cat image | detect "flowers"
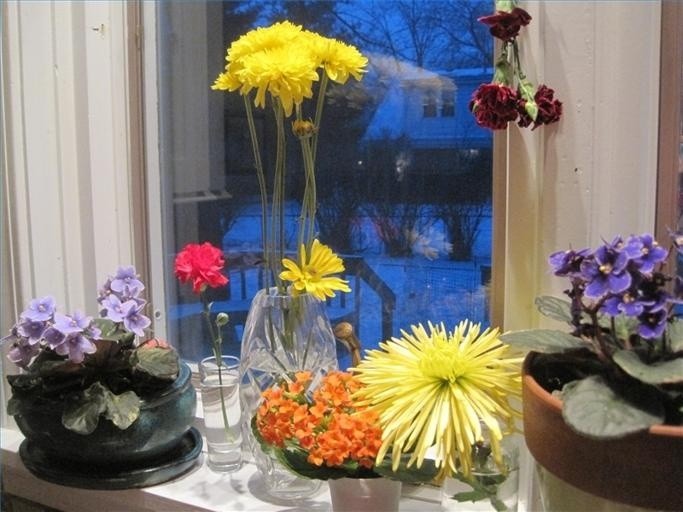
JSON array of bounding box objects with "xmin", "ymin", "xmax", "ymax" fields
[
  {"xmin": 255, "ymin": 320, "xmax": 521, "ymax": 480},
  {"xmin": 5, "ymin": 263, "xmax": 180, "ymax": 433},
  {"xmin": 211, "ymin": 18, "xmax": 371, "ymax": 383},
  {"xmin": 463, "ymin": 0, "xmax": 563, "ymax": 132},
  {"xmin": 498, "ymin": 217, "xmax": 682, "ymax": 442},
  {"xmin": 175, "ymin": 241, "xmax": 243, "ymax": 456}
]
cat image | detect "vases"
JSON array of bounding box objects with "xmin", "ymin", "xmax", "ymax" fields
[
  {"xmin": 242, "ymin": 283, "xmax": 340, "ymax": 394},
  {"xmin": 522, "ymin": 366, "xmax": 681, "ymax": 511},
  {"xmin": 9, "ymin": 357, "xmax": 193, "ymax": 454},
  {"xmin": 330, "ymin": 476, "xmax": 402, "ymax": 511},
  {"xmin": 440, "ymin": 416, "xmax": 519, "ymax": 510}
]
